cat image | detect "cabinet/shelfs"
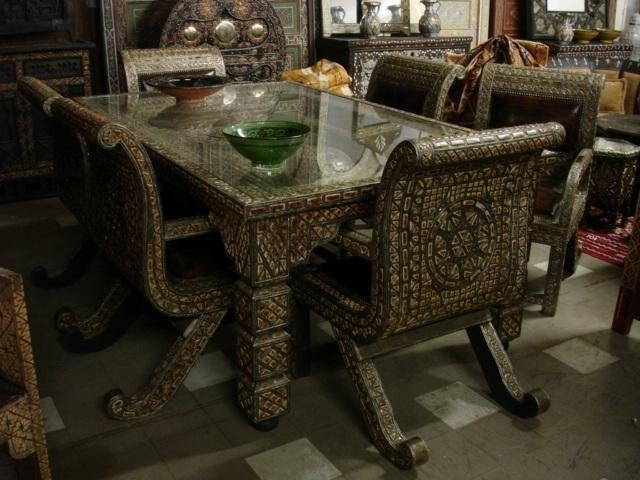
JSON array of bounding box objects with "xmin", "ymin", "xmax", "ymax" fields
[
  {"xmin": 316, "ymin": 38, "xmax": 473, "ymax": 98},
  {"xmin": 0, "ymin": 38, "xmax": 93, "ymax": 183}
]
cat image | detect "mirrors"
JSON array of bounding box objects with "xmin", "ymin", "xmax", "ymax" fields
[{"xmin": 319, "ymin": 0, "xmax": 411, "ymax": 37}]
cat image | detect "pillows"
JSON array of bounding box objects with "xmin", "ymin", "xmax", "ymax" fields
[{"xmin": 600, "ymin": 78, "xmax": 627, "ymax": 113}]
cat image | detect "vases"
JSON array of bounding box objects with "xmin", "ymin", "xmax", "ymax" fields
[
  {"xmin": 332, "ymin": 7, "xmax": 345, "ymax": 23},
  {"xmin": 557, "ymin": 15, "xmax": 574, "ymax": 46},
  {"xmin": 386, "ymin": 6, "xmax": 402, "ymax": 23},
  {"xmin": 419, "ymin": 0, "xmax": 441, "ymax": 37},
  {"xmin": 361, "ymin": 2, "xmax": 380, "ymax": 39}
]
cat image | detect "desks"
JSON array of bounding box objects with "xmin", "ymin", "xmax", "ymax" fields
[{"xmin": 544, "ymin": 41, "xmax": 634, "ymax": 60}]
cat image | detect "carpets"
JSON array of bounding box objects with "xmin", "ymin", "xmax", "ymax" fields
[{"xmin": 539, "ymin": 190, "xmax": 635, "ymax": 266}]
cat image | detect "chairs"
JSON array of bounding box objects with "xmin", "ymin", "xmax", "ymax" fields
[
  {"xmin": 285, "ymin": 122, "xmax": 565, "ymax": 471},
  {"xmin": 612, "ymin": 191, "xmax": 640, "ymax": 335},
  {"xmin": 0, "ymin": 267, "xmax": 52, "ymax": 479},
  {"xmin": 19, "ymin": 45, "xmax": 605, "ymax": 432}
]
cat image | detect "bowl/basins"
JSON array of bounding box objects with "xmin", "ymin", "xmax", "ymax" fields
[
  {"xmin": 573, "ymin": 28, "xmax": 621, "ymax": 45},
  {"xmin": 146, "ymin": 75, "xmax": 231, "ymax": 104},
  {"xmin": 223, "ymin": 120, "xmax": 312, "ymax": 169}
]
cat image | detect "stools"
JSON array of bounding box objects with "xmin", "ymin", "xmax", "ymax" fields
[{"xmin": 586, "ymin": 137, "xmax": 640, "ymax": 227}]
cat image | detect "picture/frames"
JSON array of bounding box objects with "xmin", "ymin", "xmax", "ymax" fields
[{"xmin": 526, "ymin": 0, "xmax": 609, "ymax": 39}]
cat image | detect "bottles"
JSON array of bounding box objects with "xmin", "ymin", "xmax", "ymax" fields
[
  {"xmin": 556, "ymin": 12, "xmax": 575, "ymax": 46},
  {"xmin": 419, "ymin": 0, "xmax": 441, "ymax": 39},
  {"xmin": 330, "ymin": 6, "xmax": 345, "ymax": 25},
  {"xmin": 361, "ymin": 1, "xmax": 382, "ymax": 40},
  {"xmin": 386, "ymin": 5, "xmax": 403, "ymax": 24}
]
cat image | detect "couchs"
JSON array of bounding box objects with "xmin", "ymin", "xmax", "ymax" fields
[{"xmin": 548, "ymin": 56, "xmax": 640, "ymax": 116}]
